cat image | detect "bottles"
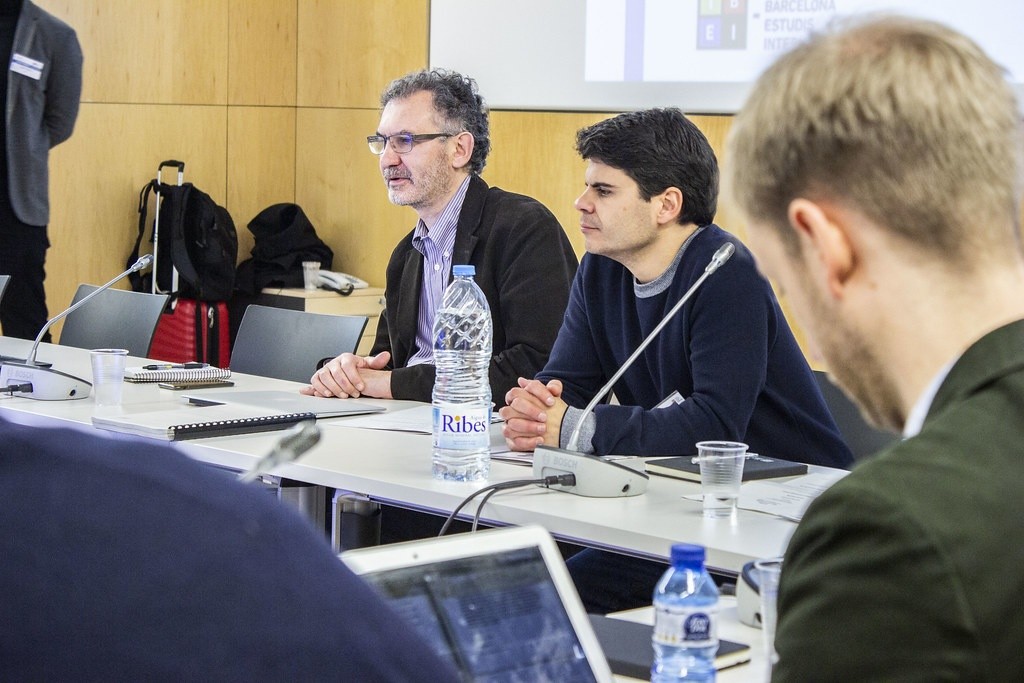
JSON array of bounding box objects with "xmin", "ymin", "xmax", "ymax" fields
[
  {"xmin": 432, "ymin": 265, "xmax": 491, "ymax": 483},
  {"xmin": 651, "ymin": 544, "xmax": 719, "ymax": 683}
]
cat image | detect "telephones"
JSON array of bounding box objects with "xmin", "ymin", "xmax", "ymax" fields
[{"xmin": 311, "ymin": 270, "xmax": 369, "ymax": 290}]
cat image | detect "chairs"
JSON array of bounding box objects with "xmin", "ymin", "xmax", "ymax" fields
[
  {"xmin": 230, "ymin": 305, "xmax": 370, "ymax": 390},
  {"xmin": 58, "ymin": 284, "xmax": 171, "ymax": 358}
]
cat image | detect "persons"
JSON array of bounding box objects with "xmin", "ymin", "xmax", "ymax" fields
[
  {"xmin": 498, "ymin": 108, "xmax": 858, "ymax": 617},
  {"xmin": 0, "ymin": 415, "xmax": 458, "ymax": 683},
  {"xmin": 0, "ymin": 0, "xmax": 83, "ymax": 345},
  {"xmin": 299, "ymin": 67, "xmax": 579, "ymax": 545},
  {"xmin": 727, "ymin": 13, "xmax": 1024, "ymax": 683}
]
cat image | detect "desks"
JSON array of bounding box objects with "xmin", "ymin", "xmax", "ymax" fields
[
  {"xmin": 0, "ymin": 336, "xmax": 852, "ymax": 580},
  {"xmin": 262, "ymin": 287, "xmax": 390, "ymax": 356},
  {"xmin": 602, "ymin": 595, "xmax": 764, "ymax": 683}
]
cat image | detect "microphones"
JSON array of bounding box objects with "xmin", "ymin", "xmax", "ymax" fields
[
  {"xmin": 0, "ymin": 254, "xmax": 157, "ymax": 400},
  {"xmin": 534, "ymin": 243, "xmax": 736, "ymax": 497}
]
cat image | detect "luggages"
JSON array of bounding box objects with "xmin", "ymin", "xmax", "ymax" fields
[{"xmin": 146, "ymin": 160, "xmax": 229, "ymax": 373}]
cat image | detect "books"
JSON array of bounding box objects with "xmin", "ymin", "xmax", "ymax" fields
[
  {"xmin": 587, "ymin": 614, "xmax": 752, "ymax": 682},
  {"xmin": 125, "ymin": 360, "xmax": 235, "ymax": 383},
  {"xmin": 644, "ymin": 455, "xmax": 808, "ymax": 485},
  {"xmin": 92, "ymin": 402, "xmax": 316, "ymax": 442}
]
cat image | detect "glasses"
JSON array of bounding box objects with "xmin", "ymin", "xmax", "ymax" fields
[{"xmin": 367, "ymin": 133, "xmax": 453, "ymax": 155}]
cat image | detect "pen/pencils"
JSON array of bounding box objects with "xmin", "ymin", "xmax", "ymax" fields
[
  {"xmin": 691, "ymin": 452, "xmax": 759, "ymax": 464},
  {"xmin": 143, "ymin": 363, "xmax": 211, "ymax": 370}
]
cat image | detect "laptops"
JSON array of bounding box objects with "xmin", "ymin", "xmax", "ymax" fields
[{"xmin": 336, "ymin": 523, "xmax": 615, "ymax": 683}]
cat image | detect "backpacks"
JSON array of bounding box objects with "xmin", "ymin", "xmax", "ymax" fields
[{"xmin": 127, "ymin": 179, "xmax": 236, "ymax": 303}]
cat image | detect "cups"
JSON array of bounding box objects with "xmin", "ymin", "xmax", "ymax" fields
[
  {"xmin": 302, "ymin": 262, "xmax": 320, "ymax": 294},
  {"xmin": 491, "ymin": 402, "xmax": 495, "ymax": 416},
  {"xmin": 90, "ymin": 349, "xmax": 129, "ymax": 406},
  {"xmin": 696, "ymin": 441, "xmax": 749, "ymax": 518},
  {"xmin": 756, "ymin": 558, "xmax": 786, "ymax": 667}
]
